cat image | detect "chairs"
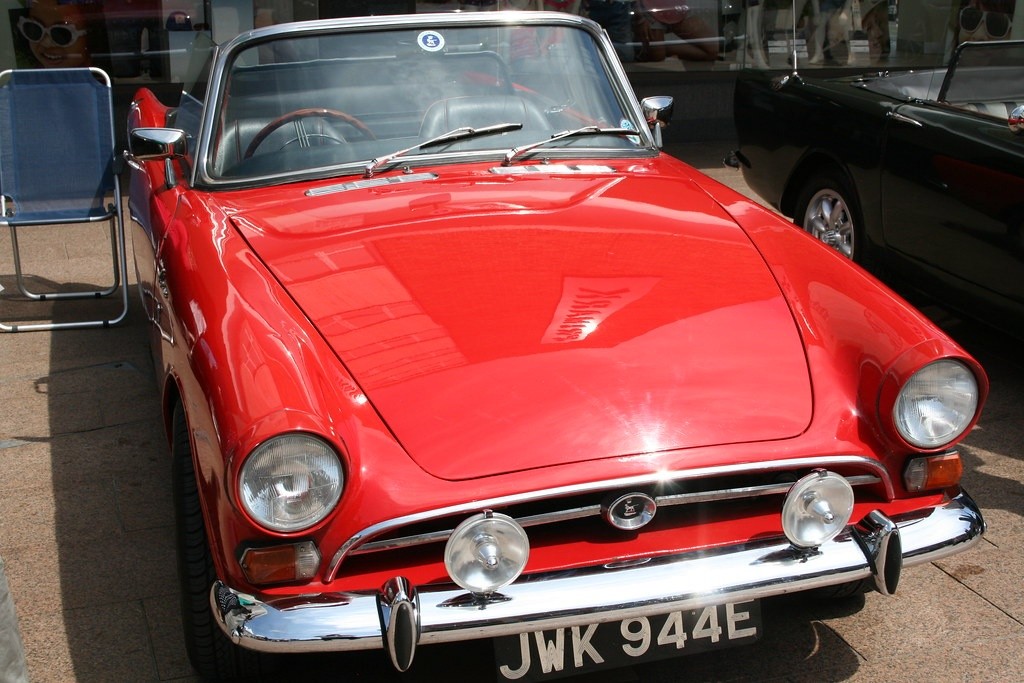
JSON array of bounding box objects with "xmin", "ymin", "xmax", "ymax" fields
[
  {"xmin": 414, "ymin": 95, "xmax": 558, "ymax": 150},
  {"xmin": 213, "ymin": 118, "xmax": 353, "ymax": 175},
  {"xmin": 0, "ymin": 66, "xmax": 129, "ymax": 334}
]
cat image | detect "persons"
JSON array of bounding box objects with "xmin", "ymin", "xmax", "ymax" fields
[
  {"xmin": 951, "ymin": 0, "xmax": 1016, "ymax": 48},
  {"xmin": 416, "ymin": 0, "xmax": 720, "ymax": 63},
  {"xmin": 17, "ymin": 0, "xmax": 90, "ymax": 69},
  {"xmin": 859, "ymin": 0, "xmax": 887, "ymax": 67},
  {"xmin": 736, "ymin": 0, "xmax": 846, "ymax": 70}
]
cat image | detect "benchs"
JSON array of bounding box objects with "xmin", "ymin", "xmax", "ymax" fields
[
  {"xmin": 961, "ymin": 101, "xmax": 1024, "ymax": 119},
  {"xmin": 186, "ymin": 84, "xmax": 538, "ymax": 139}
]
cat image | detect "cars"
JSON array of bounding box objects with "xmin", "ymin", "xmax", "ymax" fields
[
  {"xmin": 127, "ymin": 10, "xmax": 990, "ymax": 682},
  {"xmin": 725, "ymin": 39, "xmax": 1024, "ymax": 348}
]
